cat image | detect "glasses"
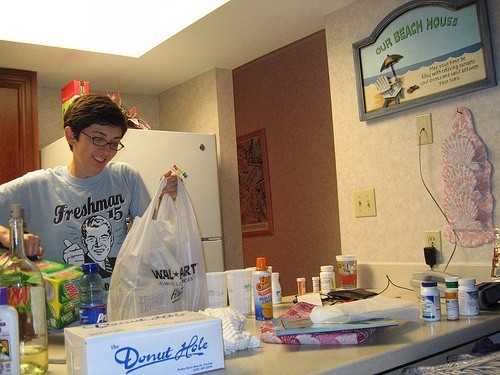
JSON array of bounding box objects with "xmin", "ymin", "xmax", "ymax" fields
[{"xmin": 80, "ymin": 131, "xmax": 124, "ymax": 151}]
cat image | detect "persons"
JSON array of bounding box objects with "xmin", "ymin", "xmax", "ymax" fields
[{"xmin": 0, "ymin": 94, "xmax": 181, "ymax": 334}]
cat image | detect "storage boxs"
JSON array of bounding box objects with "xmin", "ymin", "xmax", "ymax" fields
[{"xmin": 64, "ymin": 310, "xmax": 226, "ymax": 375}]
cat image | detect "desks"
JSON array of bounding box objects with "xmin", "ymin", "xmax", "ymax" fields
[{"xmin": 68, "ymin": 282, "xmax": 500, "ymax": 375}]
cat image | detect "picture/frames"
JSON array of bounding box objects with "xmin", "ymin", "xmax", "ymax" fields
[
  {"xmin": 351, "ymin": 0, "xmax": 500, "ymax": 121},
  {"xmin": 237, "ymin": 128, "xmax": 274, "ymax": 237}
]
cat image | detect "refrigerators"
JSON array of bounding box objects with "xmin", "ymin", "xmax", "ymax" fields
[{"xmin": 40, "ymin": 130, "xmax": 224, "ymax": 273}]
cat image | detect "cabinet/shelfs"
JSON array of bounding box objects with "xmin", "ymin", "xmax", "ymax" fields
[{"xmin": 40, "ymin": 129, "xmax": 226, "ymax": 273}]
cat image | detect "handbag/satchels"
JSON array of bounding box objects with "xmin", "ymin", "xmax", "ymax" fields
[{"xmin": 107, "ymin": 174, "xmax": 209, "ymax": 319}]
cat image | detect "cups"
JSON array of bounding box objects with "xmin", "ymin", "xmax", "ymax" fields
[{"xmin": 206, "ymin": 266, "xmax": 273, "ymax": 317}]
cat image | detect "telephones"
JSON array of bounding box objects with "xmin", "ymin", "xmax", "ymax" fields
[{"xmin": 410, "ymin": 271, "xmax": 464, "ymax": 298}]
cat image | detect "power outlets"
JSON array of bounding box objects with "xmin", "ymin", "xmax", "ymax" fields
[
  {"xmin": 415, "ymin": 113, "xmax": 434, "ymax": 146},
  {"xmin": 425, "ymin": 231, "xmax": 443, "ymax": 264}
]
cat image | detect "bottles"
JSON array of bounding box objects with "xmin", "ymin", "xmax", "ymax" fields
[
  {"xmin": 0, "ymin": 203, "xmax": 49, "ymax": 375},
  {"xmin": 311, "ymin": 277, "xmax": 320, "ymax": 293},
  {"xmin": 445, "ymin": 278, "xmax": 480, "ymax": 320},
  {"xmin": 297, "ymin": 278, "xmax": 306, "ymax": 295},
  {"xmin": 79, "ymin": 263, "xmax": 108, "ymax": 325},
  {"xmin": 272, "ymin": 273, "xmax": 282, "ymax": 304},
  {"xmin": 336, "ymin": 255, "xmax": 358, "ymax": 289},
  {"xmin": 254, "ymin": 257, "xmax": 272, "ymax": 321},
  {"xmin": 320, "ymin": 266, "xmax": 336, "ymax": 292},
  {"xmin": 421, "ymin": 280, "xmax": 441, "ymax": 322}
]
